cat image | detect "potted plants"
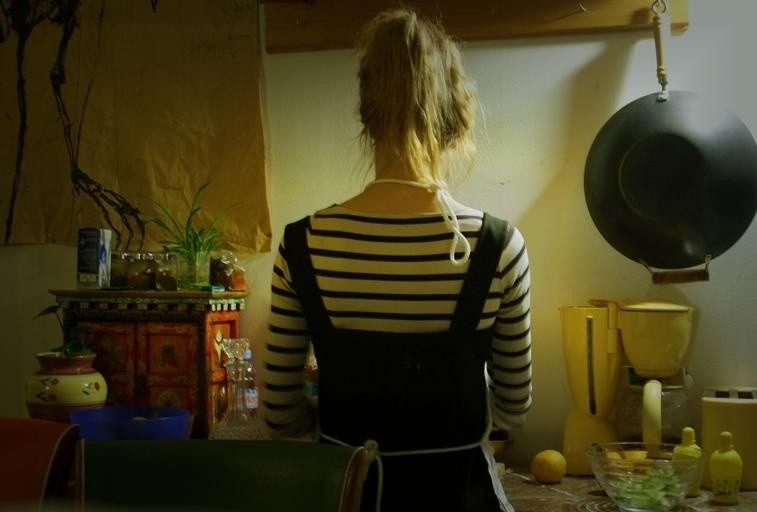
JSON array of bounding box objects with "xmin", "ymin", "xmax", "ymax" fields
[
  {"xmin": 128, "ymin": 180, "xmax": 243, "ymax": 291},
  {"xmin": 25, "ymin": 303, "xmax": 109, "ymax": 423}
]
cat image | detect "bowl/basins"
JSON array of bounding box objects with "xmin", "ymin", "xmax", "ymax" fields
[
  {"xmin": 71, "ymin": 406, "xmax": 189, "ymax": 441},
  {"xmin": 586, "ymin": 441, "xmax": 706, "ymax": 511}
]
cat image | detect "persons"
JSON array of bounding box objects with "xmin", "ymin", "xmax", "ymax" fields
[{"xmin": 258, "ymin": 6, "xmax": 535, "ymax": 511}]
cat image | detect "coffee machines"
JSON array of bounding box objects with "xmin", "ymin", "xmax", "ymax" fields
[{"xmin": 557, "ymin": 301, "xmax": 694, "ymax": 476}]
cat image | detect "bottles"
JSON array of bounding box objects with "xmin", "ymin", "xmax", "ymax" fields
[
  {"xmin": 709, "ymin": 433, "xmax": 743, "ymax": 503},
  {"xmin": 673, "ymin": 426, "xmax": 703, "ymax": 497},
  {"xmin": 112, "ymin": 251, "xmax": 179, "ymax": 292},
  {"xmin": 243, "ymin": 351, "xmax": 259, "ymax": 418}
]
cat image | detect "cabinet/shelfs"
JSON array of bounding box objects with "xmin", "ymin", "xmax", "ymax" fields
[{"xmin": 51, "ymin": 286, "xmax": 249, "ymax": 439}]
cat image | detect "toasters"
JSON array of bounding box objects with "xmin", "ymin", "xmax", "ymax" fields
[{"xmin": 702, "ymin": 387, "xmax": 756, "ymax": 493}]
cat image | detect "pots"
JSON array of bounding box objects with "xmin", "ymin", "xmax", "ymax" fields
[{"xmin": 581, "ymin": 2, "xmax": 756, "ymax": 287}]
